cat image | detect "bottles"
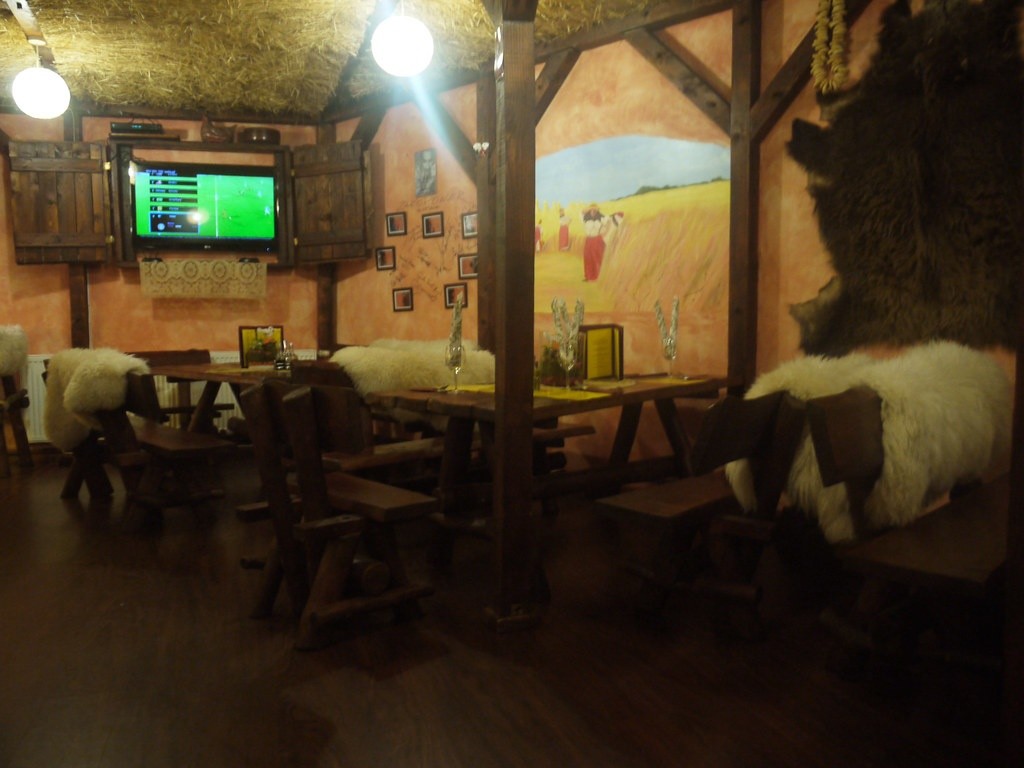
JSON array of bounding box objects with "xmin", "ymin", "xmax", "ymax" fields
[{"xmin": 275, "ymin": 341, "xmax": 299, "ymax": 370}]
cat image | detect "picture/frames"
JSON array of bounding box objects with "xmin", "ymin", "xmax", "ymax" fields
[
  {"xmin": 239, "ymin": 324, "xmax": 284, "ymax": 369},
  {"xmin": 458, "ymin": 254, "xmax": 478, "ymax": 279},
  {"xmin": 422, "ymin": 212, "xmax": 444, "ymax": 238},
  {"xmin": 461, "ymin": 211, "xmax": 478, "ymax": 238},
  {"xmin": 386, "ymin": 212, "xmax": 407, "ymax": 236},
  {"xmin": 392, "ymin": 288, "xmax": 413, "ymax": 312},
  {"xmin": 413, "ymin": 147, "xmax": 437, "ymax": 198},
  {"xmin": 376, "ymin": 246, "xmax": 395, "ymax": 270},
  {"xmin": 444, "ymin": 283, "xmax": 468, "ymax": 308}
]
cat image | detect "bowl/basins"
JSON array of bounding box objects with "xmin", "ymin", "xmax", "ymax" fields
[{"xmin": 242, "ymin": 127, "xmax": 281, "ymax": 145}]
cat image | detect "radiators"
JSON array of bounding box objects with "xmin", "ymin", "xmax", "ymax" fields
[{"xmin": 20, "ymin": 349, "xmax": 317, "ymax": 444}]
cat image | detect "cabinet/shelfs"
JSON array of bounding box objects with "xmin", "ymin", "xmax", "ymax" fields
[{"xmin": 7, "ymin": 139, "xmax": 369, "ymax": 268}]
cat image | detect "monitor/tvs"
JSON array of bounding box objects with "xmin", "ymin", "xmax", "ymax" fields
[{"xmin": 130, "ymin": 160, "xmax": 278, "ymax": 253}]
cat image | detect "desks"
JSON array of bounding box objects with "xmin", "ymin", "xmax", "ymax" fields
[
  {"xmin": 151, "ymin": 363, "xmax": 291, "ymax": 499},
  {"xmin": 364, "ymin": 373, "xmax": 728, "ymax": 577}
]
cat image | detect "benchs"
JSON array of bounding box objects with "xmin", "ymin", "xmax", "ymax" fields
[{"xmin": 0, "ymin": 323, "xmax": 1017, "ymax": 681}]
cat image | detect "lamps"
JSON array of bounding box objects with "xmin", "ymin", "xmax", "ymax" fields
[
  {"xmin": 12, "ymin": 36, "xmax": 70, "ymax": 119},
  {"xmin": 370, "ymin": 1, "xmax": 436, "ymax": 77}
]
